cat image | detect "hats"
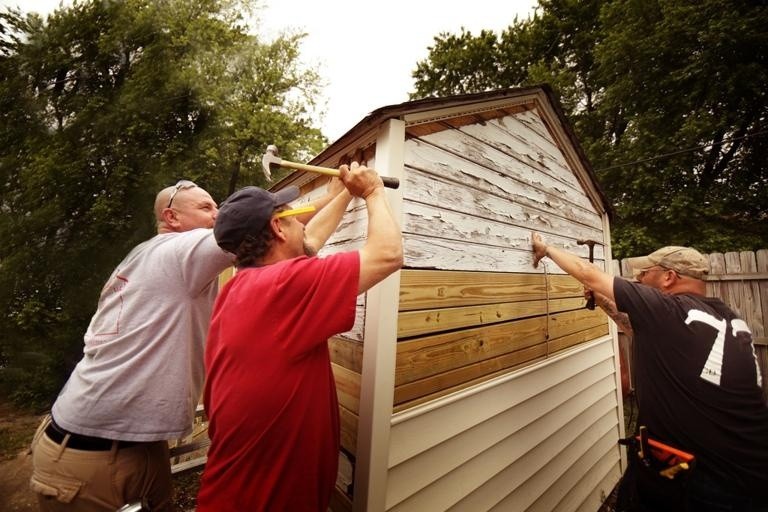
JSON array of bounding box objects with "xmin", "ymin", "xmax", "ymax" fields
[
  {"xmin": 628, "ymin": 244, "xmax": 712, "ymax": 282},
  {"xmin": 214, "ymin": 185, "xmax": 301, "ymax": 253}
]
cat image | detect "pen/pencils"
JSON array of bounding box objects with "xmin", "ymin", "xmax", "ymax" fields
[{"xmin": 271, "ymin": 206, "xmax": 317, "ymax": 219}]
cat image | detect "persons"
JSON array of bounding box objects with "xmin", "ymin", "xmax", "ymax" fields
[
  {"xmin": 29, "ymin": 164, "xmax": 349, "ymax": 512},
  {"xmin": 532, "ymin": 233, "xmax": 768, "ymax": 512},
  {"xmin": 194, "ymin": 160, "xmax": 402, "ymax": 512}
]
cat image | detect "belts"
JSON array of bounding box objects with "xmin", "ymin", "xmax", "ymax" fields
[{"xmin": 44, "ymin": 421, "xmax": 140, "ymax": 451}]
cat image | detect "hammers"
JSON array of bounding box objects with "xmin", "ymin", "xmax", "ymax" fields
[
  {"xmin": 575, "ymin": 237, "xmax": 607, "ymax": 311},
  {"xmin": 261, "ymin": 143, "xmax": 400, "ymax": 190}
]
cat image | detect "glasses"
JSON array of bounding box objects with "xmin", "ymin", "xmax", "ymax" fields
[{"xmin": 168, "ymin": 178, "xmax": 200, "ymax": 210}]
